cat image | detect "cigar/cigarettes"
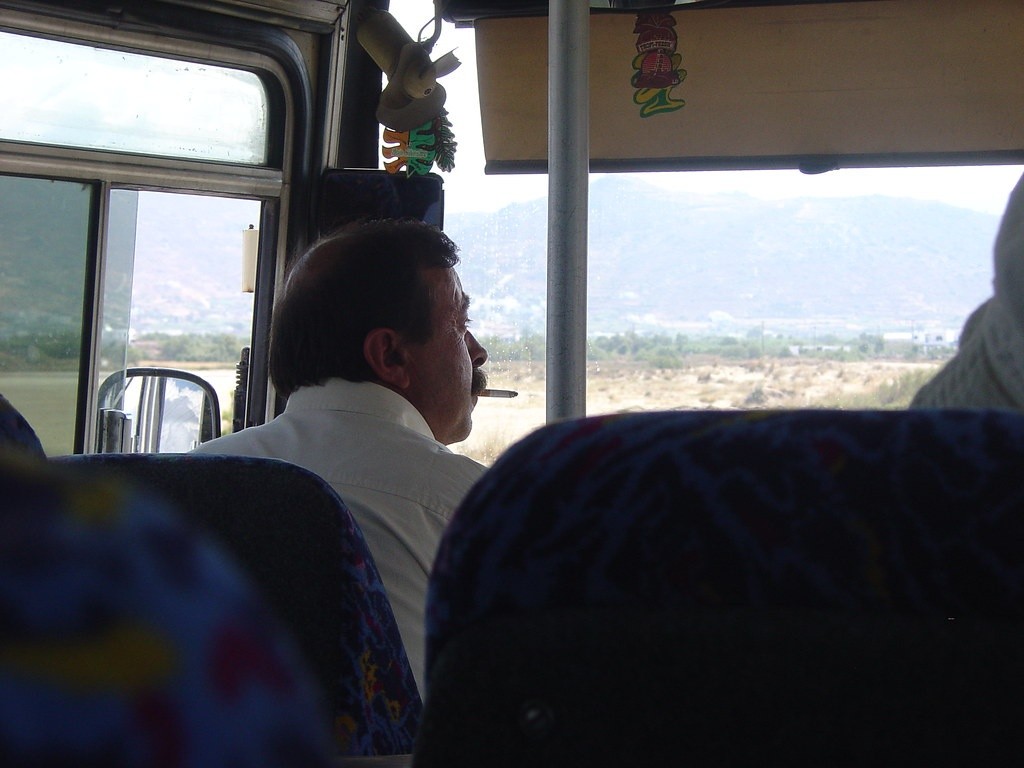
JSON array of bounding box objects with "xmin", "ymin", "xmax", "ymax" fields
[{"xmin": 479, "ymin": 388, "xmax": 518, "ymax": 398}]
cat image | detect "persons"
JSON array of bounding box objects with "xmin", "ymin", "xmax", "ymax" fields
[{"xmin": 185, "ymin": 217, "xmax": 493, "ymax": 707}]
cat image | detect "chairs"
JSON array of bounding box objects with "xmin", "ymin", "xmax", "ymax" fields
[
  {"xmin": 51, "ymin": 452, "xmax": 422, "ymax": 758},
  {"xmin": 416, "ymin": 409, "xmax": 1024, "ymax": 768}
]
